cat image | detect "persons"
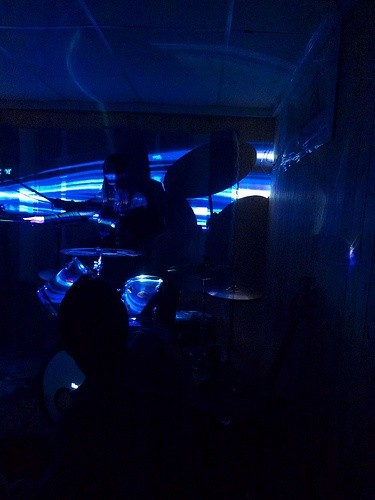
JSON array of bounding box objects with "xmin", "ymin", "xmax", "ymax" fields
[
  {"xmin": 54, "ymin": 276, "xmax": 191, "ymax": 500},
  {"xmin": 51, "ymin": 151, "xmax": 165, "ymax": 252}
]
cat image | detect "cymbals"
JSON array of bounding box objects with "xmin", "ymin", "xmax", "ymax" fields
[
  {"xmin": 57, "ymin": 245, "xmax": 142, "ymax": 258},
  {"xmin": 165, "ymin": 141, "xmax": 257, "ymax": 199},
  {"xmin": 203, "ymin": 281, "xmax": 262, "ymax": 304}
]
